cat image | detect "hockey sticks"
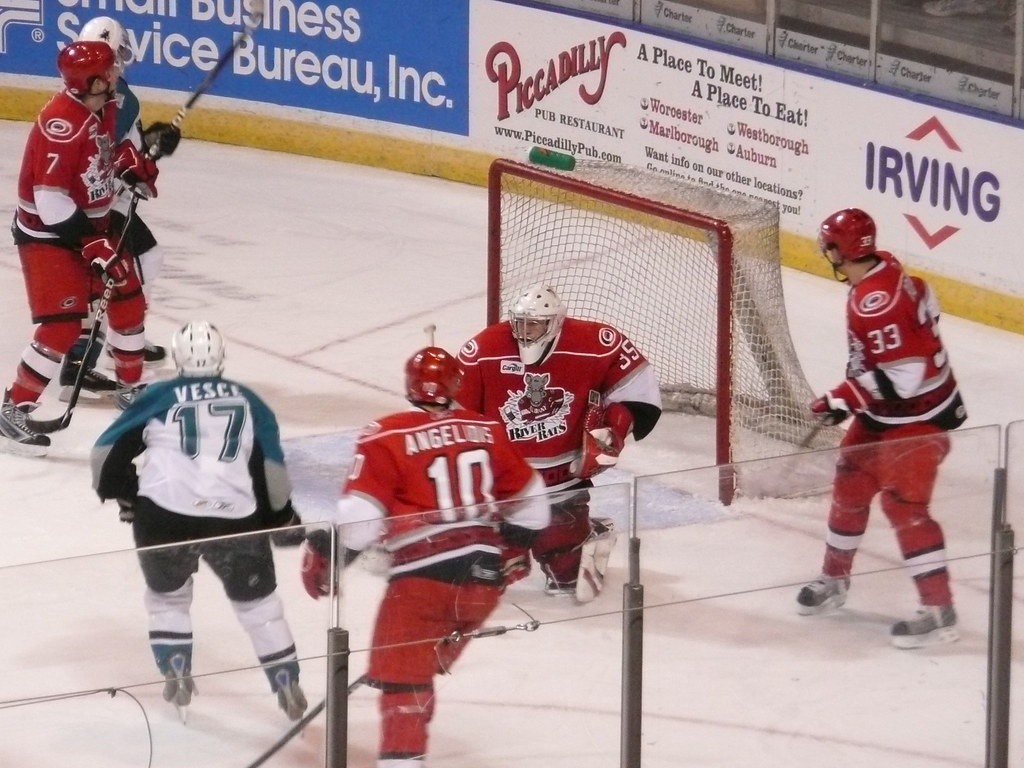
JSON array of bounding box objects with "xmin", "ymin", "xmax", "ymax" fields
[
  {"xmin": 145, "ymin": 0, "xmax": 264, "ymax": 162},
  {"xmin": 246, "ymin": 673, "xmax": 368, "ymax": 768},
  {"xmin": 732, "ymin": 414, "xmax": 829, "ymax": 494},
  {"xmin": 421, "ymin": 322, "xmax": 440, "ymax": 348},
  {"xmin": 25, "ymin": 194, "xmax": 142, "ymax": 435}
]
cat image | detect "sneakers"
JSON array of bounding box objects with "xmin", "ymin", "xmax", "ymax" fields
[
  {"xmin": 111, "ymin": 381, "xmax": 148, "ymax": 410},
  {"xmin": 143, "ymin": 343, "xmax": 166, "ymax": 361},
  {"xmin": 0, "ymin": 400, "xmax": 50, "ymax": 459},
  {"xmin": 889, "ymin": 605, "xmax": 957, "ymax": 651},
  {"xmin": 162, "ymin": 672, "xmax": 193, "ymax": 724},
  {"xmin": 797, "ymin": 576, "xmax": 850, "ymax": 614},
  {"xmin": 279, "ymin": 684, "xmax": 307, "ymax": 738},
  {"xmin": 57, "ymin": 364, "xmax": 118, "ymax": 404}
]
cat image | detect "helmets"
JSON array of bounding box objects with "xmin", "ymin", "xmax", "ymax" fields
[
  {"xmin": 78, "ymin": 16, "xmax": 135, "ymax": 67},
  {"xmin": 508, "ymin": 285, "xmax": 564, "ymax": 365},
  {"xmin": 406, "ymin": 348, "xmax": 461, "ymax": 406},
  {"xmin": 58, "ymin": 41, "xmax": 117, "ymax": 99},
  {"xmin": 171, "ymin": 321, "xmax": 225, "ymax": 380},
  {"xmin": 819, "ymin": 208, "xmax": 877, "ymax": 262}
]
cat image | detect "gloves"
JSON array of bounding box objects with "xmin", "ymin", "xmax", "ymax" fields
[
  {"xmin": 85, "ymin": 240, "xmax": 132, "ymax": 283},
  {"xmin": 269, "ymin": 503, "xmax": 306, "ymax": 547},
  {"xmin": 811, "ymin": 377, "xmax": 869, "ymax": 427},
  {"xmin": 146, "ymin": 121, "xmax": 181, "ymax": 156},
  {"xmin": 119, "ymin": 495, "xmax": 134, "ymax": 520},
  {"xmin": 300, "ymin": 529, "xmax": 337, "ymax": 602}
]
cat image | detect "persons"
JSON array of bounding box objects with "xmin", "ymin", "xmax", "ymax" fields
[
  {"xmin": 302, "ymin": 347, "xmax": 549, "ymax": 768},
  {"xmin": 795, "ymin": 209, "xmax": 968, "ymax": 648},
  {"xmin": 0, "ymin": 15, "xmax": 180, "ymax": 456},
  {"xmin": 456, "ymin": 288, "xmax": 662, "ymax": 604},
  {"xmin": 89, "ymin": 322, "xmax": 308, "ymax": 721}
]
